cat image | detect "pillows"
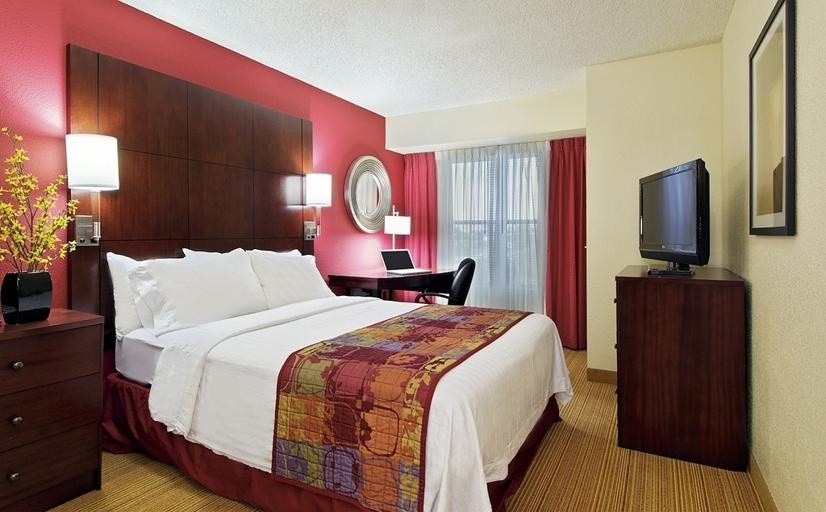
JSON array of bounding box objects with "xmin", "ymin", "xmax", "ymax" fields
[
  {"xmin": 246, "ymin": 250, "xmax": 335, "ymax": 311},
  {"xmin": 127, "ymin": 247, "xmax": 267, "ymax": 337},
  {"xmin": 107, "ymin": 252, "xmax": 144, "ymax": 341},
  {"xmin": 182, "ymin": 247, "xmax": 302, "ymax": 258}
]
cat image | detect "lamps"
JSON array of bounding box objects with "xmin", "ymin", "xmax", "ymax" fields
[
  {"xmin": 278, "ymin": 173, "xmax": 307, "ymax": 209},
  {"xmin": 384, "ymin": 204, "xmax": 411, "ymax": 250},
  {"xmin": 303, "ymin": 172, "xmax": 331, "ymax": 240},
  {"xmin": 64, "ymin": 133, "xmax": 120, "ymax": 192}
]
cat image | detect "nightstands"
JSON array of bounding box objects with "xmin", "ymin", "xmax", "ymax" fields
[{"xmin": 0, "ymin": 308, "xmax": 105, "ymax": 512}]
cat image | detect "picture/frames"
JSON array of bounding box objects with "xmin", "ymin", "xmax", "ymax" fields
[{"xmin": 747, "ymin": 0, "xmax": 796, "ymax": 236}]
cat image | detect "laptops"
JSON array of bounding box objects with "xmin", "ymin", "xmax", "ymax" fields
[{"xmin": 379, "ymin": 249, "xmax": 432, "ymax": 275}]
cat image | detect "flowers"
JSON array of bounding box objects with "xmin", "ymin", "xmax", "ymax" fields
[{"xmin": 0, "ymin": 126, "xmax": 79, "ymax": 270}]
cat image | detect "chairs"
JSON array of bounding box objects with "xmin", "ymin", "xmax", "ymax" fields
[{"xmin": 415, "ymin": 258, "xmax": 475, "ymax": 305}]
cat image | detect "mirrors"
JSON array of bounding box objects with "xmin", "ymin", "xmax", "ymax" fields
[{"xmin": 344, "ymin": 155, "xmax": 391, "ymax": 234}]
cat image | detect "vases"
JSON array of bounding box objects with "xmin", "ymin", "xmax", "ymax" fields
[{"xmin": 1, "ymin": 272, "xmax": 53, "ymax": 323}]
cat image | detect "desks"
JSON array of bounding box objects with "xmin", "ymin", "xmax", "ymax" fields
[
  {"xmin": 612, "ymin": 264, "xmax": 750, "ymax": 472},
  {"xmin": 327, "ymin": 268, "xmax": 453, "ymax": 300}
]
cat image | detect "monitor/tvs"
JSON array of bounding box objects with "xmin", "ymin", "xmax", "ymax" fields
[{"xmin": 638, "ymin": 158, "xmax": 710, "ymax": 276}]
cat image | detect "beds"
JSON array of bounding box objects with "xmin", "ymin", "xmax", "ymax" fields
[{"xmin": 96, "ymin": 254, "xmax": 574, "ymax": 511}]
